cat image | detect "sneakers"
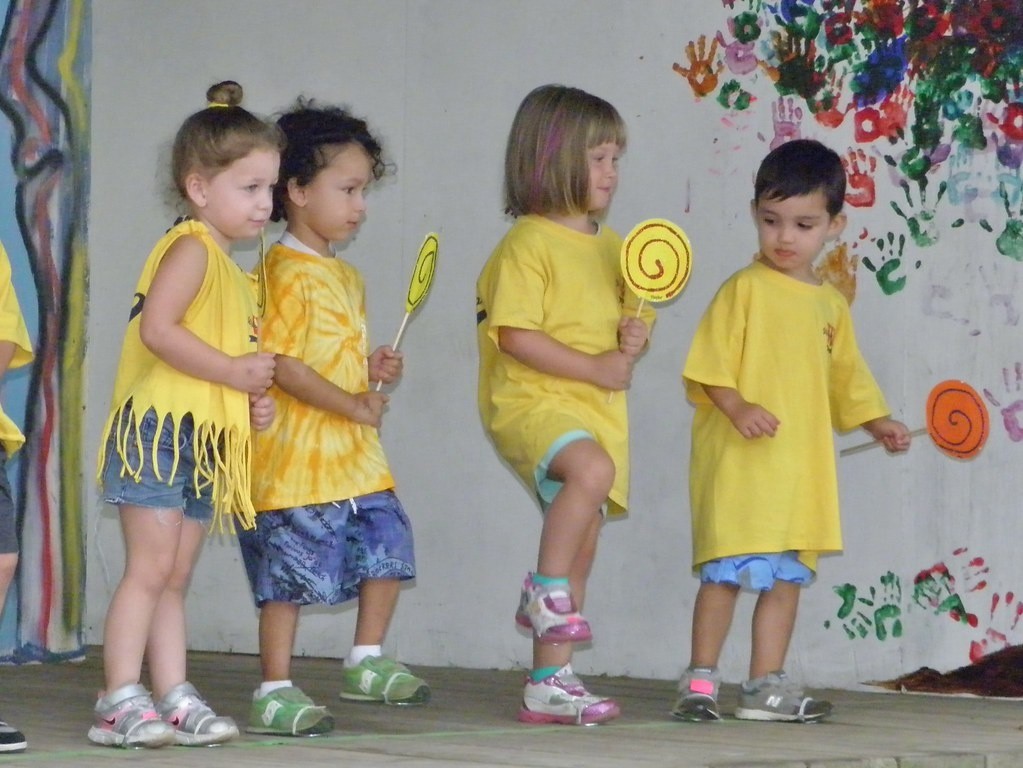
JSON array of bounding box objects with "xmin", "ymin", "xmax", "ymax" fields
[
  {"xmin": 734, "ymin": 669, "xmax": 832, "ymax": 721},
  {"xmin": 246, "ymin": 685, "xmax": 335, "ymax": 737},
  {"xmin": 518, "ymin": 671, "xmax": 621, "ymax": 724},
  {"xmin": 513, "ymin": 568, "xmax": 593, "ymax": 644},
  {"xmin": 148, "ymin": 681, "xmax": 239, "ymax": 745},
  {"xmin": 336, "ymin": 654, "xmax": 431, "ymax": 706},
  {"xmin": 87, "ymin": 683, "xmax": 176, "ymax": 747},
  {"xmin": 0, "ymin": 719, "xmax": 28, "ymax": 751},
  {"xmin": 669, "ymin": 668, "xmax": 721, "ymax": 720}
]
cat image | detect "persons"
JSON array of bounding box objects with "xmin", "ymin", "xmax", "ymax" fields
[
  {"xmin": 0, "ymin": 244, "xmax": 35, "ymax": 753},
  {"xmin": 671, "ymin": 140, "xmax": 911, "ymax": 722},
  {"xmin": 231, "ymin": 107, "xmax": 430, "ymax": 738},
  {"xmin": 85, "ymin": 80, "xmax": 288, "ymax": 750},
  {"xmin": 475, "ymin": 84, "xmax": 658, "ymax": 727}
]
